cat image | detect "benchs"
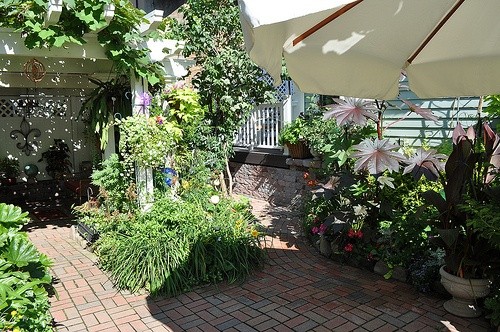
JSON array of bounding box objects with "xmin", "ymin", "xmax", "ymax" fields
[{"xmin": 59, "ymin": 161, "xmax": 100, "ymax": 206}]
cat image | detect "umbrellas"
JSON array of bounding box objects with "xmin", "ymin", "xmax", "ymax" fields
[{"xmin": 240, "ymin": 0, "xmax": 500, "ymax": 101}]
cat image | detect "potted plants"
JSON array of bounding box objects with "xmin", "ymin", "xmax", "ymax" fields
[
  {"xmin": 0, "ymin": 157, "xmax": 21, "ymax": 185},
  {"xmin": 417, "ymin": 121, "xmax": 500, "ymax": 318},
  {"xmin": 277, "ymin": 116, "xmax": 313, "ymax": 158}
]
